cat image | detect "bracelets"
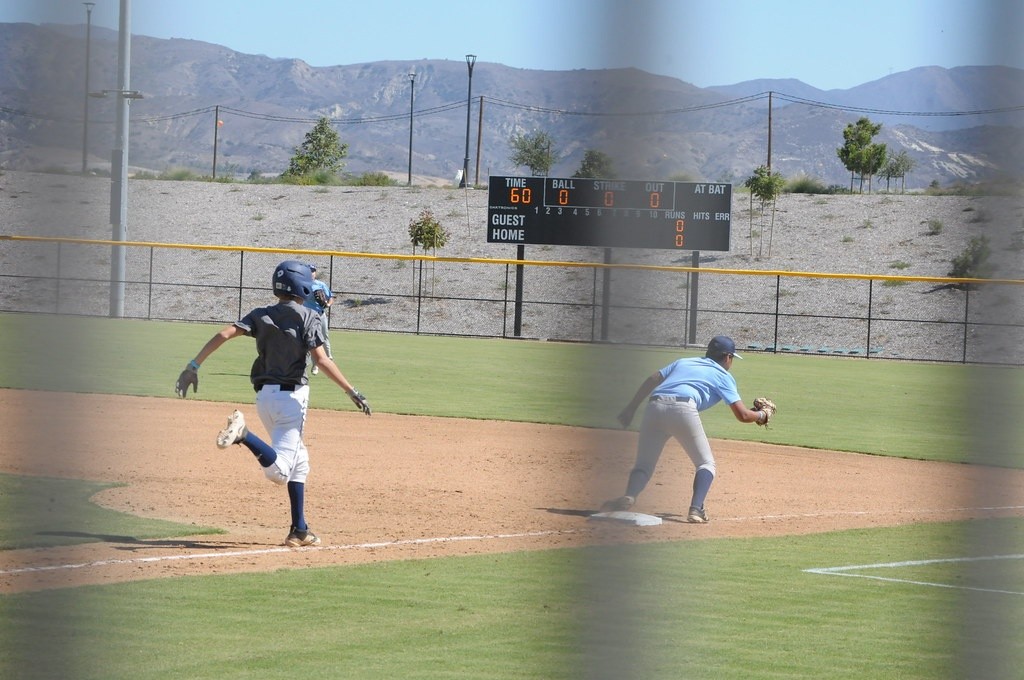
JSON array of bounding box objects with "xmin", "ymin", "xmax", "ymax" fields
[
  {"xmin": 757, "ymin": 411, "xmax": 763, "ymax": 423},
  {"xmin": 190, "ymin": 360, "xmax": 201, "ymax": 369}
]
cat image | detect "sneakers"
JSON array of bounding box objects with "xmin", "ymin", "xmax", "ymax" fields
[
  {"xmin": 285, "ymin": 523, "xmax": 321, "ymax": 547},
  {"xmin": 600, "ymin": 495, "xmax": 635, "ymax": 513},
  {"xmin": 216, "ymin": 408, "xmax": 248, "ymax": 449},
  {"xmin": 687, "ymin": 503, "xmax": 710, "ymax": 523}
]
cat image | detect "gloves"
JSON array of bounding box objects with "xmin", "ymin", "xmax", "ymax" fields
[
  {"xmin": 175, "ymin": 359, "xmax": 201, "ymax": 399},
  {"xmin": 345, "ymin": 387, "xmax": 372, "ymax": 417}
]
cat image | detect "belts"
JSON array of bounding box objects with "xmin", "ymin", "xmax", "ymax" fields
[{"xmin": 649, "ymin": 395, "xmax": 691, "ymax": 402}]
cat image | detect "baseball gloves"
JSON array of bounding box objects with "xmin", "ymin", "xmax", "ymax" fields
[
  {"xmin": 313, "ymin": 288, "xmax": 327, "ymax": 309},
  {"xmin": 749, "ymin": 396, "xmax": 777, "ymax": 429}
]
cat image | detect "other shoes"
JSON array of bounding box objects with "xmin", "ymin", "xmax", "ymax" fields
[{"xmin": 311, "ymin": 366, "xmax": 319, "ymax": 375}]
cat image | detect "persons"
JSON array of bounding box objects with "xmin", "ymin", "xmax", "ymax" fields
[
  {"xmin": 598, "ymin": 335, "xmax": 777, "ymax": 524},
  {"xmin": 301, "ymin": 263, "xmax": 333, "ymax": 376},
  {"xmin": 176, "ymin": 260, "xmax": 372, "ymax": 548}
]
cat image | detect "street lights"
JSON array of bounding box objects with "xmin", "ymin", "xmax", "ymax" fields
[
  {"xmin": 408, "ymin": 64, "xmax": 418, "ymax": 186},
  {"xmin": 81, "ymin": 0, "xmax": 97, "ymax": 174},
  {"xmin": 458, "ymin": 53, "xmax": 477, "ymax": 189}
]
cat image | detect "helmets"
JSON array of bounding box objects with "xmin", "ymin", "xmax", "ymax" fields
[{"xmin": 272, "ymin": 259, "xmax": 313, "ymax": 301}]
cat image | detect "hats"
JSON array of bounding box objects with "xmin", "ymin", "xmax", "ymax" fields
[
  {"xmin": 308, "ymin": 264, "xmax": 317, "ymax": 269},
  {"xmin": 708, "ymin": 335, "xmax": 744, "ymax": 360}
]
